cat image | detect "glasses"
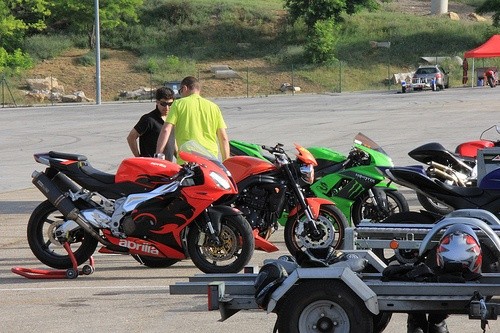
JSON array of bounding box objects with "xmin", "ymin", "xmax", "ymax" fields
[
  {"xmin": 159, "ymin": 99, "xmax": 173, "ymax": 106},
  {"xmin": 178, "ymin": 86, "xmax": 183, "ymax": 93}
]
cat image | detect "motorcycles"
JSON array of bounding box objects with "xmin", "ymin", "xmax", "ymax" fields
[
  {"xmin": 27, "ymin": 139, "xmax": 254, "ymax": 271},
  {"xmin": 364, "ymin": 163, "xmax": 500, "ymax": 278},
  {"xmin": 485, "ymin": 67, "xmax": 499, "ymax": 88},
  {"xmin": 227, "ymin": 134, "xmax": 407, "ymax": 245},
  {"xmin": 126, "ymin": 142, "xmax": 350, "ymax": 268}
]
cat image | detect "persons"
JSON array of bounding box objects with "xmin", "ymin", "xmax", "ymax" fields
[
  {"xmin": 153, "ymin": 76, "xmax": 231, "ymax": 162},
  {"xmin": 126, "ymin": 87, "xmax": 178, "ymax": 162}
]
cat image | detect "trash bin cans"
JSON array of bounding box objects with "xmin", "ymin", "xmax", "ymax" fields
[{"xmin": 165, "ymin": 81, "xmax": 181, "ymax": 98}]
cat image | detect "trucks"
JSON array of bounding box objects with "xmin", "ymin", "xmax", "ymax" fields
[{"xmin": 209, "ymin": 208, "xmax": 500, "ymax": 333}]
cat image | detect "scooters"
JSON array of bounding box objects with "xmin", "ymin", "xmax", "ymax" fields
[{"xmin": 375, "ymin": 125, "xmax": 499, "ymax": 218}]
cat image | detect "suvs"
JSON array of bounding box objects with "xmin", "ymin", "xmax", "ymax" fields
[{"xmin": 412, "ymin": 65, "xmax": 449, "ymax": 91}]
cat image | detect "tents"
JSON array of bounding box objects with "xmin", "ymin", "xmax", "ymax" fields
[{"xmin": 464, "ymin": 34, "xmax": 500, "ymax": 88}]
